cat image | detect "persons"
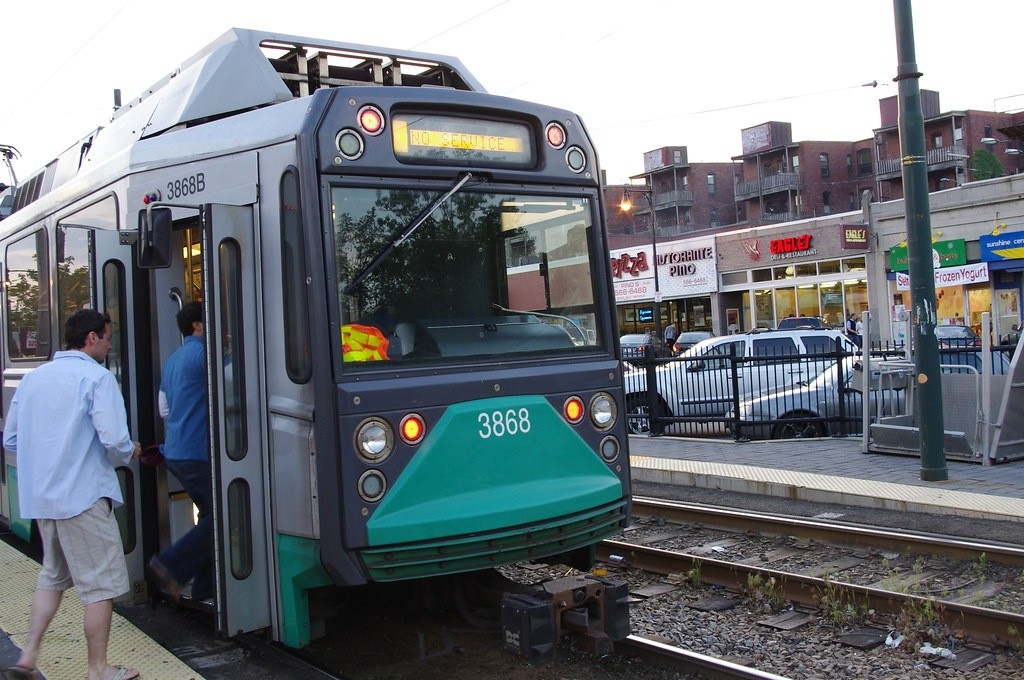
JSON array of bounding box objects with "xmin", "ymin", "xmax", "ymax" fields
[
  {"xmin": 147, "ymin": 300, "xmax": 231, "ymax": 606},
  {"xmin": 789, "ymin": 314, "xmax": 794, "ymax": 317},
  {"xmin": 728, "ymin": 318, "xmax": 739, "ymax": 335},
  {"xmin": 664, "ymin": 322, "xmax": 677, "ymax": 350},
  {"xmin": 800, "ymin": 314, "xmax": 805, "ymax": 317},
  {"xmin": 856, "ymin": 314, "xmax": 864, "ymax": 348},
  {"xmin": 3, "ymin": 308, "xmax": 144, "ymax": 680},
  {"xmin": 846, "ymin": 313, "xmax": 861, "ymax": 351}
]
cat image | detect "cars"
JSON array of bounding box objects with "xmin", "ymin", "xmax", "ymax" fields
[
  {"xmin": 726, "ymin": 346, "xmax": 1009, "ymax": 438},
  {"xmin": 1001, "ymin": 320, "xmax": 1024, "ymax": 362},
  {"xmin": 620, "ymin": 333, "xmax": 672, "ymax": 358},
  {"xmin": 748, "ymin": 327, "xmax": 774, "ymax": 332},
  {"xmin": 936, "ymin": 325, "xmax": 981, "ymax": 349},
  {"xmin": 673, "ymin": 331, "xmax": 718, "ymax": 358}
]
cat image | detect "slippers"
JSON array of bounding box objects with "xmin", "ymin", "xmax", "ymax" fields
[
  {"xmin": 7, "ymin": 667, "xmax": 39, "ymax": 680},
  {"xmin": 88, "ymin": 667, "xmax": 139, "ymax": 680}
]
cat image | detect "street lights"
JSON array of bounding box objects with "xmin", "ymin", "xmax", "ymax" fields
[{"xmin": 620, "ymin": 187, "xmax": 663, "ymax": 342}]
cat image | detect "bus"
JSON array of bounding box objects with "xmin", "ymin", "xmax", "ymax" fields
[{"xmin": 0, "ymin": 27, "xmax": 641, "ymax": 661}]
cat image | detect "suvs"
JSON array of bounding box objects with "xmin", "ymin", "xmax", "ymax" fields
[
  {"xmin": 623, "ymin": 326, "xmax": 861, "ymax": 437},
  {"xmin": 777, "ymin": 315, "xmax": 833, "ymax": 330}
]
